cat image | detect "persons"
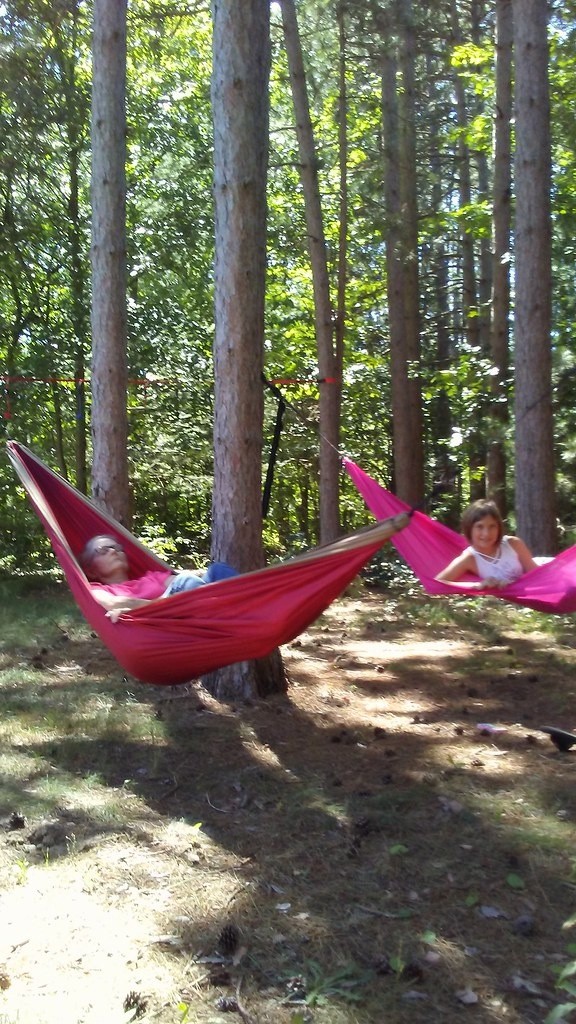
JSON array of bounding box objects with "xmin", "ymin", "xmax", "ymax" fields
[{"xmin": 82, "ymin": 499, "xmax": 540, "ymax": 624}]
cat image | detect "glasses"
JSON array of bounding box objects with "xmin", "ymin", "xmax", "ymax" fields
[{"xmin": 89, "ymin": 544, "xmax": 125, "ymax": 563}]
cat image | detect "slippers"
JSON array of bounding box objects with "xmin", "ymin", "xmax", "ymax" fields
[{"xmin": 539, "ymin": 726, "xmax": 576, "ymax": 751}]
